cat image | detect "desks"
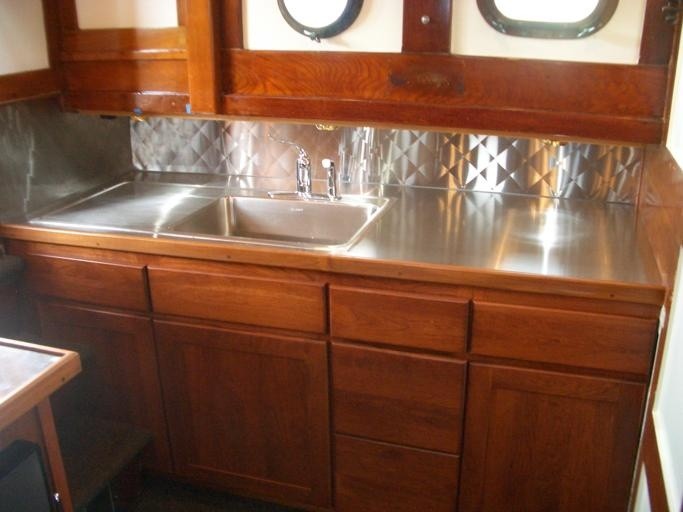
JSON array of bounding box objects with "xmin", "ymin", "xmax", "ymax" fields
[{"xmin": 0, "ymin": 337, "xmax": 82, "ymax": 512}]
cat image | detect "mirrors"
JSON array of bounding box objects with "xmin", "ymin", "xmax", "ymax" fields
[
  {"xmin": 277, "ymin": 0, "xmax": 363, "ymax": 43},
  {"xmin": 477, "ymin": 0, "xmax": 619, "ymax": 39}
]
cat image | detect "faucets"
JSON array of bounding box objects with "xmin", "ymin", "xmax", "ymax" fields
[
  {"xmin": 320, "ymin": 158, "xmax": 337, "ymax": 195},
  {"xmin": 266, "ymin": 132, "xmax": 312, "ymax": 201}
]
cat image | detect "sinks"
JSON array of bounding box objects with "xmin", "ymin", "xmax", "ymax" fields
[{"xmin": 168, "ymin": 190, "xmax": 401, "ymax": 254}]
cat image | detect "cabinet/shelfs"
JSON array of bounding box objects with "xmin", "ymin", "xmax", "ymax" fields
[
  {"xmin": 329, "ymin": 271, "xmax": 472, "ymax": 512},
  {"xmin": 29, "ymin": 249, "xmax": 328, "ymax": 512},
  {"xmin": 458, "ymin": 286, "xmax": 660, "ymax": 512}
]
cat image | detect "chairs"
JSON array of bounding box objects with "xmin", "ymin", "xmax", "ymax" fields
[{"xmin": 0, "ymin": 255, "xmax": 152, "ymax": 512}]
